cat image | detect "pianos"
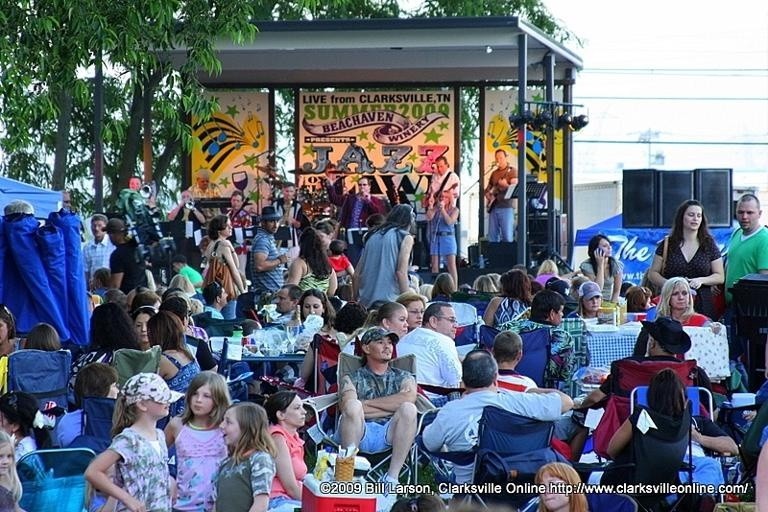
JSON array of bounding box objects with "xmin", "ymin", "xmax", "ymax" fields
[{"xmin": 193, "ymin": 198, "xmax": 232, "ymax": 207}]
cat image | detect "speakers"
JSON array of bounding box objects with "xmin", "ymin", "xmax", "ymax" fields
[
  {"xmin": 622, "ymin": 169, "xmax": 656, "ymax": 228},
  {"xmin": 659, "ymin": 170, "xmax": 695, "ymax": 229},
  {"xmin": 528, "ymin": 215, "xmax": 568, "ymax": 266},
  {"xmin": 695, "ymin": 168, "xmax": 733, "ymax": 228},
  {"xmin": 483, "ymin": 241, "xmax": 525, "ymax": 274}
]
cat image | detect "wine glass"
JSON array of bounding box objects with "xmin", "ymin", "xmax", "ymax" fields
[
  {"xmin": 253, "ymin": 329, "xmax": 265, "ymax": 357},
  {"xmin": 287, "ymin": 325, "xmax": 299, "ymax": 353}
]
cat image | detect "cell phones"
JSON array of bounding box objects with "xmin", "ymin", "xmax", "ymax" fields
[{"xmin": 597, "ymin": 248, "xmax": 604, "ymax": 255}]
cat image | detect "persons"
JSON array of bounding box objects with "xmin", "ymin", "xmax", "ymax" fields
[{"xmin": 1, "ymin": 151, "xmax": 767, "ymax": 512}]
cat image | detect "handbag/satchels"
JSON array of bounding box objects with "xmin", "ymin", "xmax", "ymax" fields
[
  {"xmin": 711, "ymin": 286, "xmax": 726, "ymax": 319},
  {"xmin": 640, "ymin": 236, "xmax": 669, "ymax": 297},
  {"xmin": 200, "ymin": 242, "xmax": 235, "ymax": 300}
]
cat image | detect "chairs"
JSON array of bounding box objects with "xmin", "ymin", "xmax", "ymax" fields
[{"xmin": 5, "ymin": 284, "xmax": 768, "ymax": 511}]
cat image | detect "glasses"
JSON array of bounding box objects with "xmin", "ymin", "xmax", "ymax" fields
[{"xmin": 438, "ymin": 314, "xmax": 457, "ymax": 324}]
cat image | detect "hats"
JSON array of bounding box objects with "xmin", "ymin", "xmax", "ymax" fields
[
  {"xmin": 640, "ymin": 315, "xmax": 692, "ymax": 355},
  {"xmin": 362, "ymin": 325, "xmax": 400, "ymax": 345},
  {"xmin": 100, "ymin": 218, "xmax": 127, "ymax": 234},
  {"xmin": 259, "ymin": 206, "xmax": 283, "ymax": 221},
  {"xmin": 120, "ymin": 372, "xmax": 186, "ymax": 405},
  {"xmin": 578, "ymin": 280, "xmax": 603, "ymax": 301}
]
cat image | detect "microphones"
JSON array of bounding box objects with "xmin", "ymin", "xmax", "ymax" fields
[
  {"xmin": 360, "ymin": 189, "xmax": 369, "ymax": 199},
  {"xmin": 267, "ymin": 151, "xmax": 275, "ymax": 160}
]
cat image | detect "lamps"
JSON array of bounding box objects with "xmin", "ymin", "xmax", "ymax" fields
[{"xmin": 509, "ymin": 101, "xmax": 589, "ymax": 132}]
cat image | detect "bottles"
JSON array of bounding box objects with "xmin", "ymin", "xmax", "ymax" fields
[
  {"xmin": 477, "ymin": 315, "xmax": 485, "ymax": 346},
  {"xmin": 294, "ymin": 304, "xmax": 303, "ymax": 332},
  {"xmin": 324, "ymin": 447, "xmax": 334, "ymax": 472},
  {"xmin": 231, "ymin": 325, "xmax": 244, "ymax": 336}
]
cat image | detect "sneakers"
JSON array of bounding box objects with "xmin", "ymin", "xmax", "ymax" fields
[{"xmin": 378, "ymin": 473, "xmax": 401, "ymax": 502}]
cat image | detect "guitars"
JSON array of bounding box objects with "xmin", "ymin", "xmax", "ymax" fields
[
  {"xmin": 422, "ymin": 183, "xmax": 458, "ymax": 208},
  {"xmin": 486, "ymin": 167, "xmax": 514, "ymax": 212}
]
